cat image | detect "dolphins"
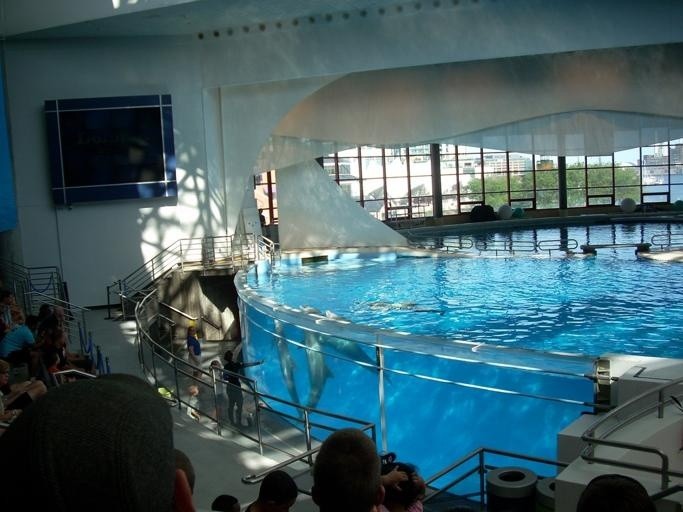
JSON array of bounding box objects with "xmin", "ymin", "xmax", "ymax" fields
[{"xmin": 272, "ymin": 303, "xmax": 393, "ymax": 419}]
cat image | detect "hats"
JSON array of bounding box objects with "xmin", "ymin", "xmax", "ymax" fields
[{"xmin": 0, "ymin": 373, "xmax": 179, "ymax": 512}]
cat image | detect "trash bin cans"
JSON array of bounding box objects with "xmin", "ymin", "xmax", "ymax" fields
[{"xmin": 486, "ymin": 466, "xmax": 556, "ymax": 512}]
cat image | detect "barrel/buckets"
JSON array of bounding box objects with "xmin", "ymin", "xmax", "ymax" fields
[{"xmin": 158, "ymin": 385, "xmax": 176, "ymax": 397}]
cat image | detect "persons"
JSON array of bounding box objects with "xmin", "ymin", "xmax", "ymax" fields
[
  {"xmin": 577, "ymin": 474, "xmax": 652, "ymax": 511},
  {"xmin": 379, "ymin": 464, "xmax": 425, "ymax": 512},
  {"xmin": 246, "ymin": 470, "xmax": 297, "ymax": 512},
  {"xmin": 223, "ymin": 351, "xmax": 264, "ymax": 426},
  {"xmin": 211, "ymin": 495, "xmax": 240, "ymax": 512},
  {"xmin": 210, "ymin": 361, "xmax": 226, "ymax": 417},
  {"xmin": 310, "ymin": 428, "xmax": 385, "ymax": 511}
]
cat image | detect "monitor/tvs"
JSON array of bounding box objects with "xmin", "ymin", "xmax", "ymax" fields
[{"xmin": 45, "ymin": 94, "xmax": 177, "ymax": 205}]
